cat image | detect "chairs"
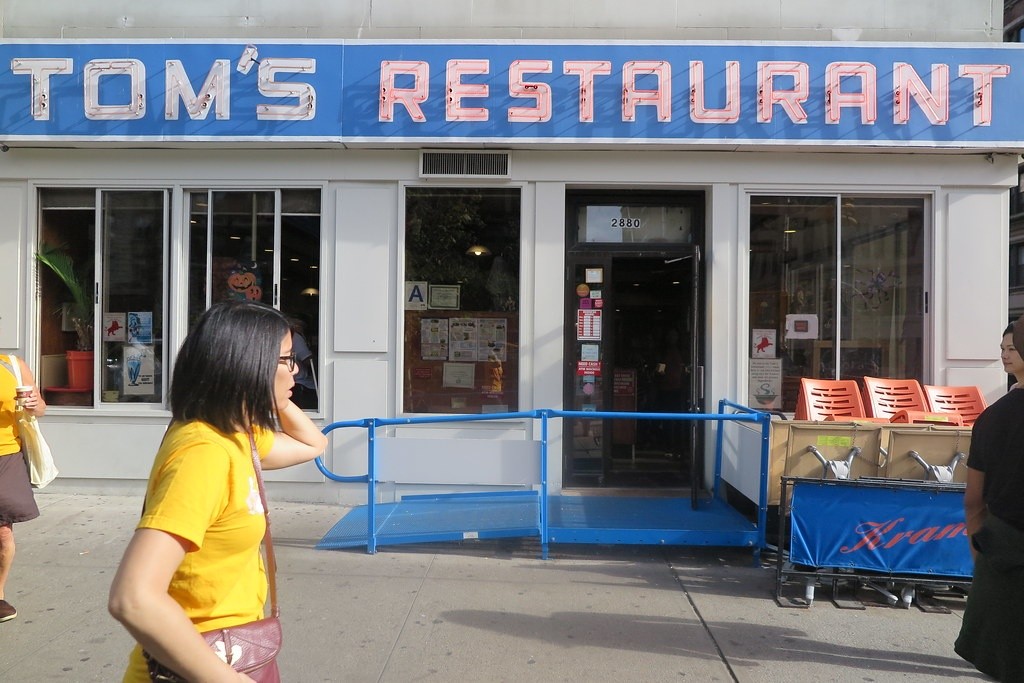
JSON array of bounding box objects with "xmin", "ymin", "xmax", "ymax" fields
[{"xmin": 793, "ymin": 377, "xmax": 987, "ymax": 428}]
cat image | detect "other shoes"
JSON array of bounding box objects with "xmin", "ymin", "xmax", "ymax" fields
[{"xmin": 0, "ymin": 600, "xmax": 17, "ymax": 623}]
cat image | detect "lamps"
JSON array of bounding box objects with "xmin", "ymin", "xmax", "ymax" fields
[{"xmin": 465, "ymin": 245, "xmax": 491, "ymax": 256}]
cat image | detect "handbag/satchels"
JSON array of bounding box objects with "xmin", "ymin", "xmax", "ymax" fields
[
  {"xmin": 143, "ymin": 609, "xmax": 283, "ymax": 683},
  {"xmin": 8, "ymin": 353, "xmax": 59, "ymax": 490}
]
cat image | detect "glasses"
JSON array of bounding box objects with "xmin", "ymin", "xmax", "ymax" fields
[{"xmin": 280, "ymin": 351, "xmax": 296, "ymax": 371}]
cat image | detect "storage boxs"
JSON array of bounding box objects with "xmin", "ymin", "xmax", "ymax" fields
[{"xmin": 739, "ymin": 413, "xmax": 971, "ymax": 504}]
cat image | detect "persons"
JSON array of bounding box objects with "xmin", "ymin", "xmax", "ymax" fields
[
  {"xmin": 479, "ymin": 343, "xmax": 504, "ymax": 406},
  {"xmin": 954, "ymin": 314, "xmax": 1024, "ymax": 683},
  {"xmin": 289, "ymin": 322, "xmax": 319, "ymax": 409},
  {"xmin": 0, "ymin": 354, "xmax": 47, "ymax": 624},
  {"xmin": 108, "ymin": 299, "xmax": 326, "ymax": 683}
]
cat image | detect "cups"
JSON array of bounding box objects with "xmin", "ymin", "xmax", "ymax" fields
[{"xmin": 16, "ymin": 385, "xmax": 33, "ymax": 407}]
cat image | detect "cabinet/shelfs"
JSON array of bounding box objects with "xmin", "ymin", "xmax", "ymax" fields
[{"xmin": 749, "ymin": 290, "xmax": 885, "ymax": 412}]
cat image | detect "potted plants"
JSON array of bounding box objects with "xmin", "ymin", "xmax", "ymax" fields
[{"xmin": 35, "ymin": 250, "xmax": 94, "ymax": 390}]
